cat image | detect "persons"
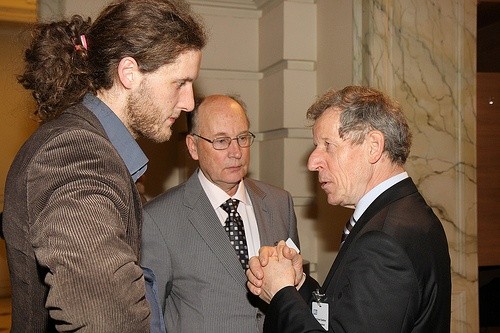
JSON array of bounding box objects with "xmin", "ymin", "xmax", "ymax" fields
[
  {"xmin": 140, "ymin": 92, "xmax": 302, "ymax": 333},
  {"xmin": 1, "ymin": 1, "xmax": 214, "ymax": 333},
  {"xmin": 244, "ymin": 84, "xmax": 453, "ymax": 333}
]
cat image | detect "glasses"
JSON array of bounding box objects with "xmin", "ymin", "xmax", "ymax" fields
[{"xmin": 191, "ymin": 131, "xmax": 255, "ymax": 150}]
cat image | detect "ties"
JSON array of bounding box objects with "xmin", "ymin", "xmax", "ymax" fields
[
  {"xmin": 220, "ymin": 198, "xmax": 249, "ymax": 275},
  {"xmin": 338, "ymin": 209, "xmax": 357, "ymax": 252}
]
